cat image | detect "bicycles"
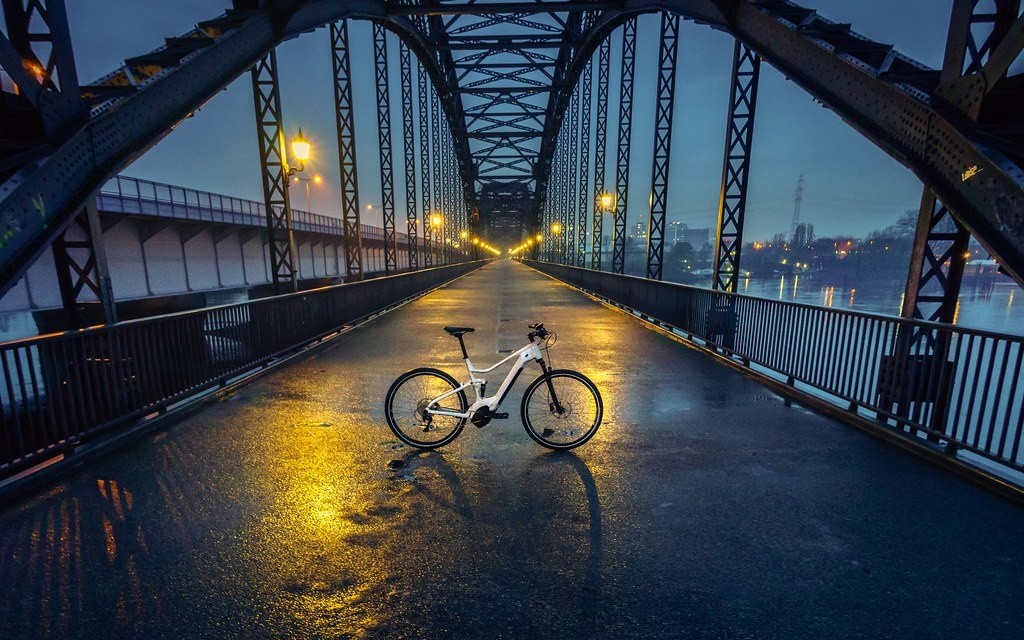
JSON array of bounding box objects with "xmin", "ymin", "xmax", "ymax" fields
[{"xmin": 385, "ymin": 322, "xmax": 604, "ymax": 453}]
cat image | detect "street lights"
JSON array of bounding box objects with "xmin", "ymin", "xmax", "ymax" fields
[{"xmin": 295, "ymin": 175, "xmax": 321, "ymax": 210}]
